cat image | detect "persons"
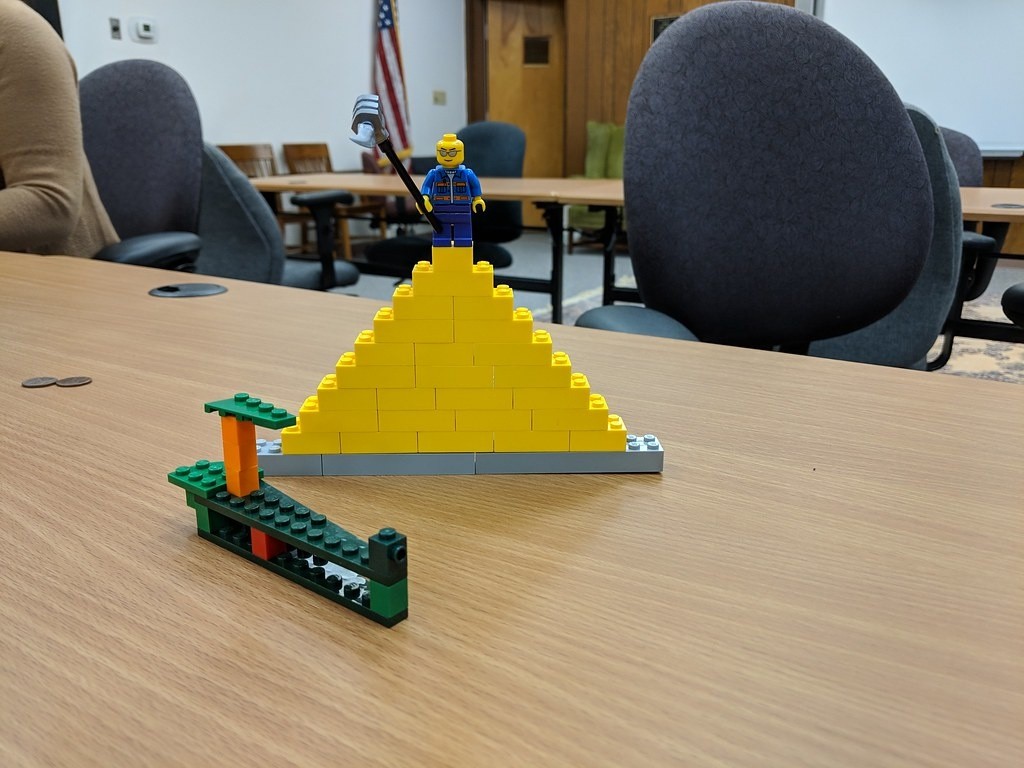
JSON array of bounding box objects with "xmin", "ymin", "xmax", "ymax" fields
[
  {"xmin": 0, "ymin": 0, "xmax": 123, "ymax": 263},
  {"xmin": 415, "ymin": 133, "xmax": 486, "ymax": 248}
]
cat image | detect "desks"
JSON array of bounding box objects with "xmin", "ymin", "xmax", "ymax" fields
[
  {"xmin": 0, "ymin": 251, "xmax": 1023, "ymax": 768},
  {"xmin": 246, "ymin": 171, "xmax": 1024, "ymax": 346}
]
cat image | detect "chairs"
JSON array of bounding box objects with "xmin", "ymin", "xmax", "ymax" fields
[
  {"xmin": 216, "ymin": 144, "xmax": 313, "ymax": 266},
  {"xmin": 78, "ymin": 59, "xmax": 203, "ymax": 276},
  {"xmin": 568, "ymin": 118, "xmax": 626, "ymax": 254},
  {"xmin": 282, "ymin": 143, "xmax": 386, "ymax": 262},
  {"xmin": 808, "ymin": 99, "xmax": 996, "ymax": 372},
  {"xmin": 457, "ymin": 121, "xmax": 526, "ymax": 267},
  {"xmin": 198, "ymin": 138, "xmax": 360, "ymax": 291},
  {"xmin": 574, "ymin": 2, "xmax": 935, "ymax": 358},
  {"xmin": 937, "ymin": 124, "xmax": 1011, "ymax": 303},
  {"xmin": 1001, "ymin": 282, "xmax": 1023, "ymax": 327}
]
